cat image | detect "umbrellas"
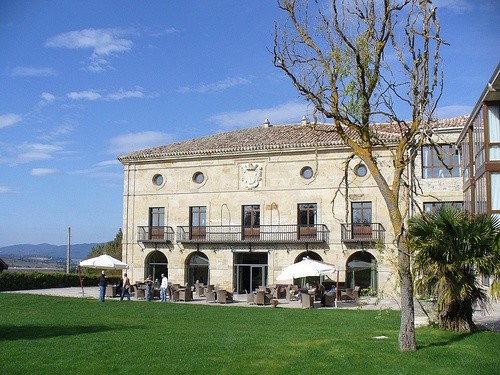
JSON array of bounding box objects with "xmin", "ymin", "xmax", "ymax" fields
[
  {"xmin": 280, "ymin": 256, "xmax": 338, "ymax": 279},
  {"xmin": 79, "ymin": 253, "xmax": 128, "ymax": 270}
]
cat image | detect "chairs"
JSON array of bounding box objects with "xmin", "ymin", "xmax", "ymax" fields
[
  {"xmin": 245, "ymin": 284, "xmax": 300, "ymax": 306},
  {"xmin": 301, "ymin": 289, "xmax": 335, "ymax": 308},
  {"xmin": 341, "ymin": 286, "xmax": 360, "ymax": 300},
  {"xmin": 131, "ymin": 281, "xmax": 236, "ymax": 304}
]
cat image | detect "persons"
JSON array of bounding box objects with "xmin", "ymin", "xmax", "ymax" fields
[
  {"xmin": 144, "ymin": 275, "xmax": 153, "ymax": 302},
  {"xmin": 97, "ymin": 270, "xmax": 108, "ymax": 302},
  {"xmin": 299, "ymin": 278, "xmax": 337, "ymax": 294},
  {"xmin": 121, "ymin": 274, "xmax": 132, "ymax": 301},
  {"xmin": 159, "ymin": 274, "xmax": 168, "ymax": 303}
]
